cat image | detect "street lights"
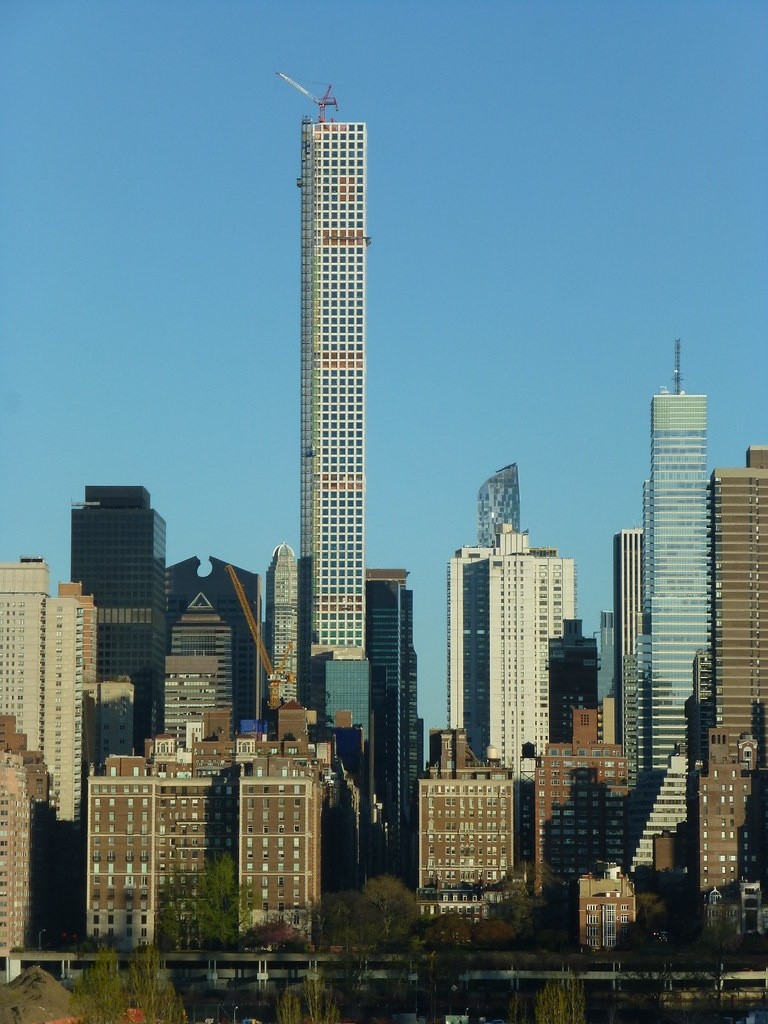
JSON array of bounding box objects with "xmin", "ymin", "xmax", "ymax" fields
[{"xmin": 38, "ymin": 929, "xmax": 46, "ymax": 951}]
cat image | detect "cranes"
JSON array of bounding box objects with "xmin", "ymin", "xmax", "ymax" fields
[
  {"xmin": 671, "ymin": 337, "xmax": 685, "ymax": 394},
  {"xmin": 275, "ymin": 71, "xmax": 340, "ymax": 123},
  {"xmin": 224, "ymin": 562, "xmax": 298, "ymax": 708}
]
